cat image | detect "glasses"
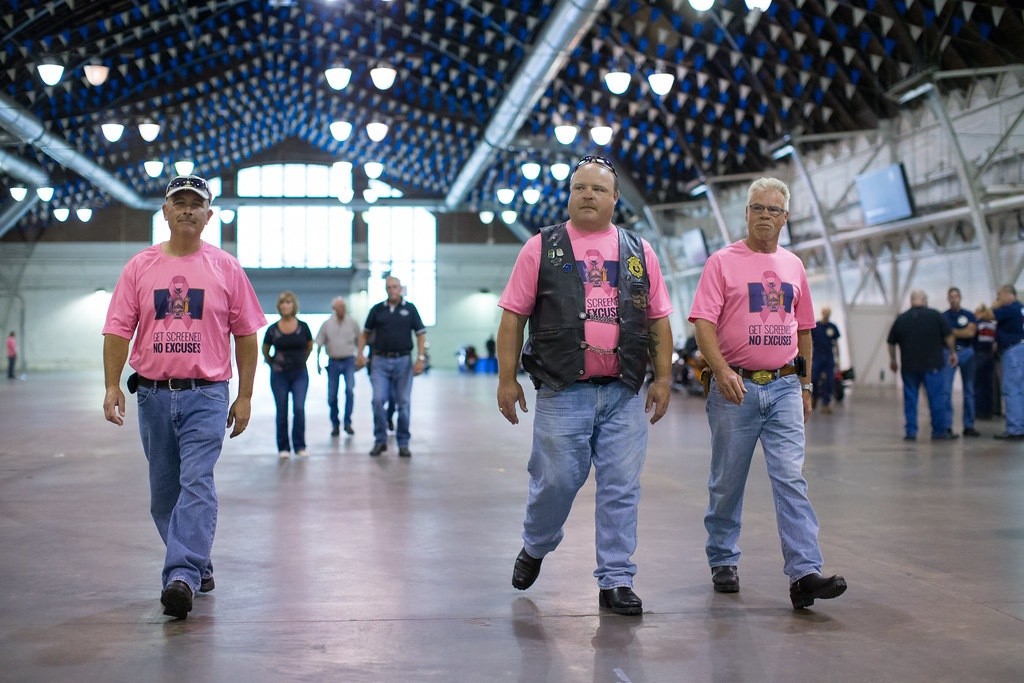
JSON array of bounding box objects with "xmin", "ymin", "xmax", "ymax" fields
[
  {"xmin": 748, "ymin": 204, "xmax": 786, "ymax": 217},
  {"xmin": 167, "ymin": 178, "xmax": 209, "ymax": 194},
  {"xmin": 575, "ymin": 155, "xmax": 618, "ymax": 178}
]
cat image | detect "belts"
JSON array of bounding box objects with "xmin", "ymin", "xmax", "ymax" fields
[
  {"xmin": 574, "ymin": 375, "xmax": 619, "ymax": 385},
  {"xmin": 332, "ymin": 356, "xmax": 354, "ymax": 361},
  {"xmin": 729, "ymin": 364, "xmax": 797, "ymax": 386},
  {"xmin": 372, "ymin": 348, "xmax": 409, "ymax": 359},
  {"xmin": 137, "ymin": 375, "xmax": 218, "ymax": 392}
]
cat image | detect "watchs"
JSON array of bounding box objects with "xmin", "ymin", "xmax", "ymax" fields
[
  {"xmin": 418, "ymin": 354, "xmax": 425, "ymax": 361},
  {"xmin": 801, "ymin": 383, "xmax": 813, "ymax": 393}
]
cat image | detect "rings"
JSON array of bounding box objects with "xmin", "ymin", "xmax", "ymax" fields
[{"xmin": 499, "ymin": 407, "xmax": 503, "ymax": 411}]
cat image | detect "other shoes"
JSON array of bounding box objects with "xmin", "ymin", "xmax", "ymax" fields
[
  {"xmin": 280, "ymin": 450, "xmax": 289, "ymax": 458},
  {"xmin": 345, "ymin": 423, "xmax": 354, "ymax": 434},
  {"xmin": 963, "ymin": 428, "xmax": 980, "ymax": 438},
  {"xmin": 389, "ymin": 420, "xmax": 393, "ymax": 431},
  {"xmin": 297, "ymin": 448, "xmax": 307, "ymax": 457},
  {"xmin": 932, "ymin": 432, "xmax": 959, "ymax": 441},
  {"xmin": 332, "ymin": 425, "xmax": 339, "ymax": 435},
  {"xmin": 993, "ymin": 430, "xmax": 1024, "ymax": 439},
  {"xmin": 400, "ymin": 447, "xmax": 410, "ymax": 457},
  {"xmin": 370, "ymin": 443, "xmax": 387, "ymax": 456},
  {"xmin": 905, "ymin": 436, "xmax": 915, "ymax": 441}
]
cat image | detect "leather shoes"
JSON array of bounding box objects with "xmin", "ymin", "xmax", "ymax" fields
[
  {"xmin": 712, "ymin": 565, "xmax": 741, "ymax": 593},
  {"xmin": 161, "ymin": 580, "xmax": 193, "ymax": 619},
  {"xmin": 512, "ymin": 546, "xmax": 543, "ymax": 591},
  {"xmin": 199, "ymin": 577, "xmax": 215, "ymax": 592},
  {"xmin": 789, "ymin": 572, "xmax": 847, "ymax": 609},
  {"xmin": 598, "ymin": 586, "xmax": 643, "ymax": 615}
]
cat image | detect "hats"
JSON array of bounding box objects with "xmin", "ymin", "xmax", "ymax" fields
[{"xmin": 165, "ymin": 175, "xmax": 212, "ymax": 207}]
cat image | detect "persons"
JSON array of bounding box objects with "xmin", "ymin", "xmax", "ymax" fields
[
  {"xmin": 811, "ymin": 307, "xmax": 840, "ymax": 414},
  {"xmin": 262, "ymin": 290, "xmax": 312, "ymax": 457},
  {"xmin": 688, "ymin": 177, "xmax": 847, "ymax": 609},
  {"xmin": 357, "ymin": 275, "xmax": 425, "ymax": 457},
  {"xmin": 102, "ymin": 174, "xmax": 267, "ymax": 618},
  {"xmin": 312, "ymin": 297, "xmax": 364, "ymax": 436},
  {"xmin": 7, "ymin": 330, "xmax": 17, "ymax": 378},
  {"xmin": 464, "ymin": 334, "xmax": 496, "ymax": 370},
  {"xmin": 496, "ymin": 155, "xmax": 674, "ymax": 614},
  {"xmin": 888, "ymin": 283, "xmax": 1024, "ymax": 442}
]
